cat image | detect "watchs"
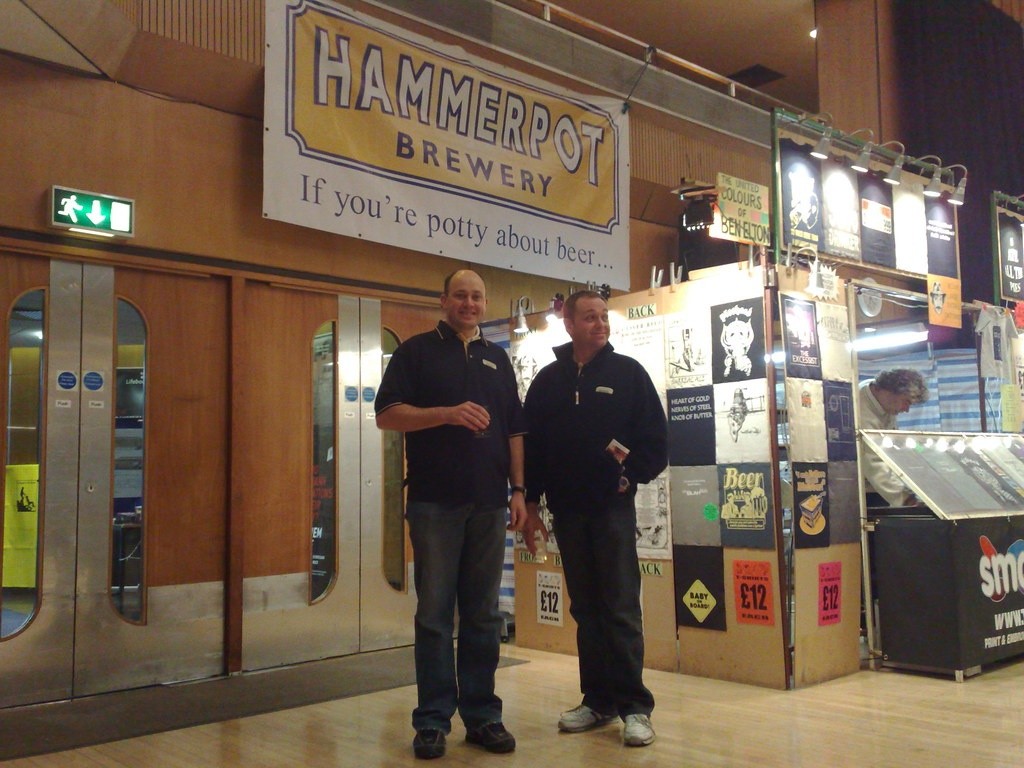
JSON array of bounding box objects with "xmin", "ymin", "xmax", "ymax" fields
[{"xmin": 618, "ymin": 477, "xmax": 630, "ymax": 491}]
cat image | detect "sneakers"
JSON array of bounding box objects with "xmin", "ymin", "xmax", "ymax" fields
[
  {"xmin": 557, "ymin": 704, "xmax": 618, "ymax": 731},
  {"xmin": 465, "ymin": 722, "xmax": 516, "ymax": 752},
  {"xmin": 624, "ymin": 713, "xmax": 657, "ymax": 746},
  {"xmin": 413, "ymin": 729, "xmax": 446, "ymax": 759}
]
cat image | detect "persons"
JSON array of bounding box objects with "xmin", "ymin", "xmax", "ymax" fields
[
  {"xmin": 522, "ymin": 291, "xmax": 668, "ymax": 746},
  {"xmin": 856, "ymin": 368, "xmax": 926, "ymax": 507},
  {"xmin": 374, "ymin": 269, "xmax": 529, "ymax": 759}
]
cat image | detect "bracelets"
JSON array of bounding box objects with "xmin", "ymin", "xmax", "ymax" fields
[{"xmin": 509, "ymin": 486, "xmax": 528, "ymax": 495}]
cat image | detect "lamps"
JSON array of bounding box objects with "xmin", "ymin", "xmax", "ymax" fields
[
  {"xmin": 799, "ymin": 112, "xmax": 968, "ymax": 206},
  {"xmin": 670, "ymin": 176, "xmax": 718, "ymax": 230}
]
